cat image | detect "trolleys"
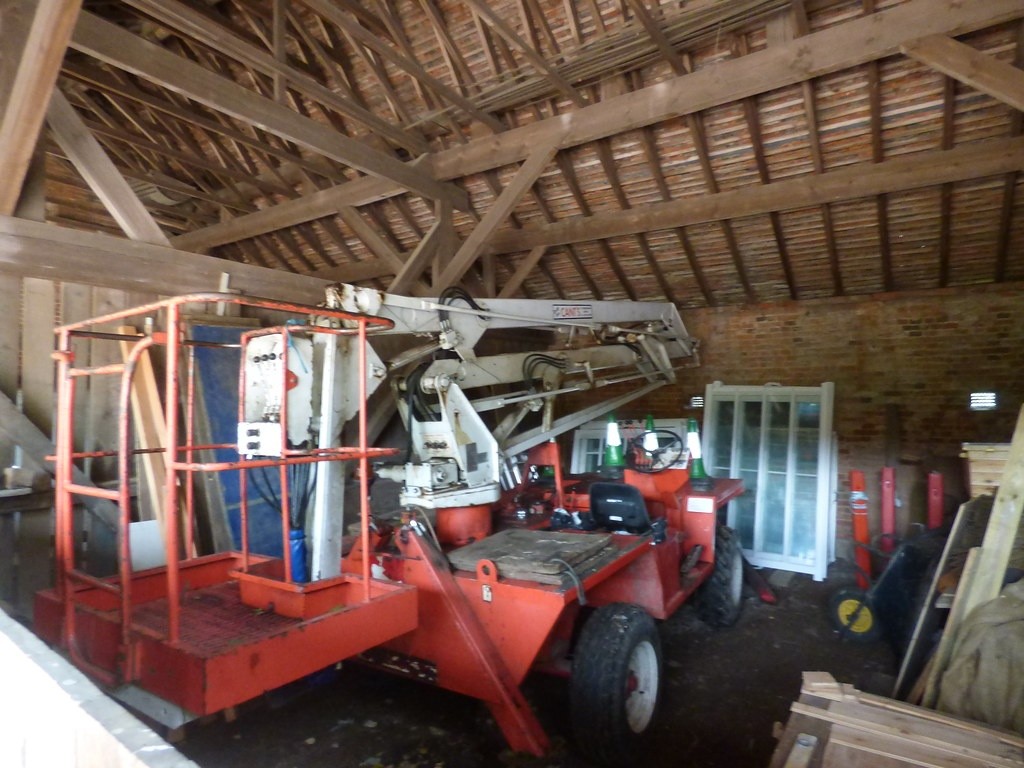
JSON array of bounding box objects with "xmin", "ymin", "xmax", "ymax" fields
[{"xmin": 822, "ymin": 532, "xmax": 914, "ymax": 641}]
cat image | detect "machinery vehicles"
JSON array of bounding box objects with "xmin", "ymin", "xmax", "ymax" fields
[{"xmin": 32, "ymin": 270, "xmax": 751, "ymax": 765}]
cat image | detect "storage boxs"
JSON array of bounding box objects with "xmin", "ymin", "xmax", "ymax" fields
[{"xmin": 958, "ymin": 443, "xmax": 1011, "ymax": 498}]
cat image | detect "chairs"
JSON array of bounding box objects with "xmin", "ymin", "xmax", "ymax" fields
[{"xmin": 590, "ymin": 482, "xmax": 651, "ymax": 533}]
